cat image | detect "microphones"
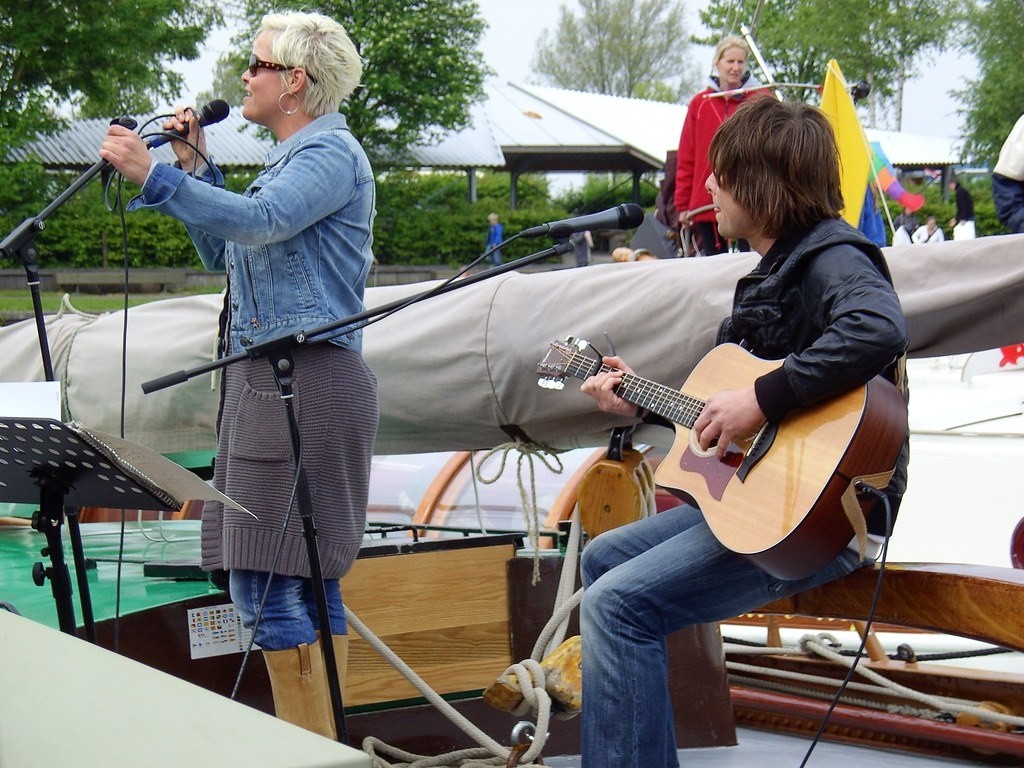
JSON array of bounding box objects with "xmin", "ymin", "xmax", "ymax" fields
[
  {"xmin": 147, "ymin": 99, "xmax": 230, "ymax": 150},
  {"xmin": 520, "ymin": 203, "xmax": 645, "ymax": 237}
]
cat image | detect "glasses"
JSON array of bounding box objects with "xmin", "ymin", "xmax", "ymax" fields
[{"xmin": 248, "ymin": 54, "xmax": 318, "ymax": 85}]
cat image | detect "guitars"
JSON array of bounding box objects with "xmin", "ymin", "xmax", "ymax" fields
[{"xmin": 532, "ymin": 331, "xmax": 907, "ymax": 575}]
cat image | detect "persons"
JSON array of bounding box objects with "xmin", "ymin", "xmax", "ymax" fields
[
  {"xmin": 992, "ymin": 114, "xmax": 1024, "ymax": 234},
  {"xmin": 949, "ymin": 184, "xmax": 974, "ymax": 228},
  {"xmin": 893, "ymin": 207, "xmax": 920, "ymax": 242},
  {"xmin": 579, "ymin": 95, "xmax": 910, "ymax": 768},
  {"xmin": 911, "ymin": 216, "xmax": 944, "ymax": 244},
  {"xmin": 100, "ymin": 10, "xmax": 380, "ymax": 739},
  {"xmin": 675, "ymin": 36, "xmax": 778, "ymax": 256},
  {"xmin": 485, "ymin": 212, "xmax": 502, "ymax": 266}
]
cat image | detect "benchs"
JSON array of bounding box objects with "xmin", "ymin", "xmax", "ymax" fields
[{"xmin": 749, "ymin": 559, "xmax": 1024, "ymax": 648}]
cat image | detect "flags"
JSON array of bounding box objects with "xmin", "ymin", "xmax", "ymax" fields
[{"xmin": 819, "ymin": 59, "xmax": 871, "ymax": 230}]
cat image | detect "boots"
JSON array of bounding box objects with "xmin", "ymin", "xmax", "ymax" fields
[{"xmin": 262, "ymin": 629, "xmax": 349, "ymax": 741}]
